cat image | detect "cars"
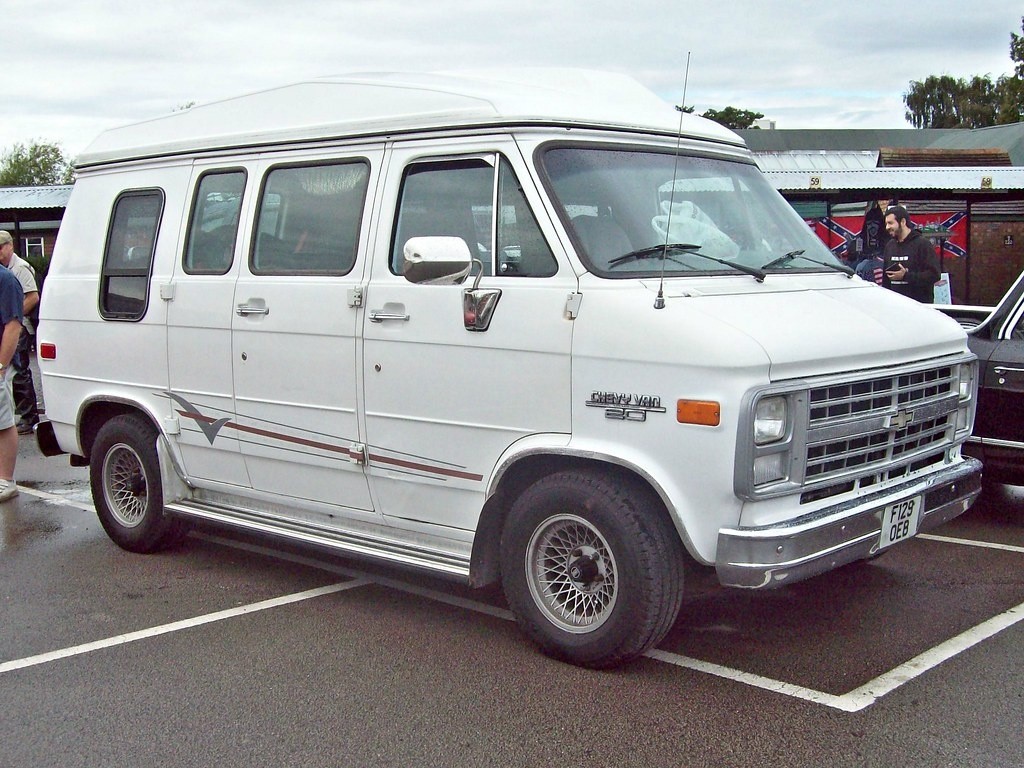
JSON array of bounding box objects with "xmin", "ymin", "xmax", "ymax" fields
[{"xmin": 963, "ymin": 267, "xmax": 1024, "ymax": 489}]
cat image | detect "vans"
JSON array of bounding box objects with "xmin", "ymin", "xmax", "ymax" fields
[{"xmin": 33, "ymin": 64, "xmax": 983, "ymax": 673}]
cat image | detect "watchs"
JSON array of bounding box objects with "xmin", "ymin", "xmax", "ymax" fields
[{"xmin": 0, "ymin": 363, "xmax": 8, "ymax": 370}]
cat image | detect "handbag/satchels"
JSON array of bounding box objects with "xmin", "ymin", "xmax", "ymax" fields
[{"xmin": 16, "ymin": 316, "xmax": 35, "ymax": 353}]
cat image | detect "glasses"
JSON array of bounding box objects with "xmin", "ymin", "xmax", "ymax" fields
[{"xmin": 0, "ymin": 243, "xmax": 7, "ymax": 250}]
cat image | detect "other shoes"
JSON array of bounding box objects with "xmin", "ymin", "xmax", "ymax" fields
[
  {"xmin": 0, "ymin": 480, "xmax": 19, "ymax": 502},
  {"xmin": 16, "ymin": 415, "xmax": 40, "ymax": 434}
]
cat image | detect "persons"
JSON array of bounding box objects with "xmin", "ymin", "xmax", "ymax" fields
[
  {"xmin": 0, "ymin": 229, "xmax": 40, "ymax": 435},
  {"xmin": 0, "ymin": 265, "xmax": 24, "ymax": 505},
  {"xmin": 881, "ymin": 207, "xmax": 942, "ymax": 304}
]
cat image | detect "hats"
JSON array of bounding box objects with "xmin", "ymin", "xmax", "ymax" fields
[{"xmin": 0, "ymin": 231, "xmax": 12, "ymax": 244}]
